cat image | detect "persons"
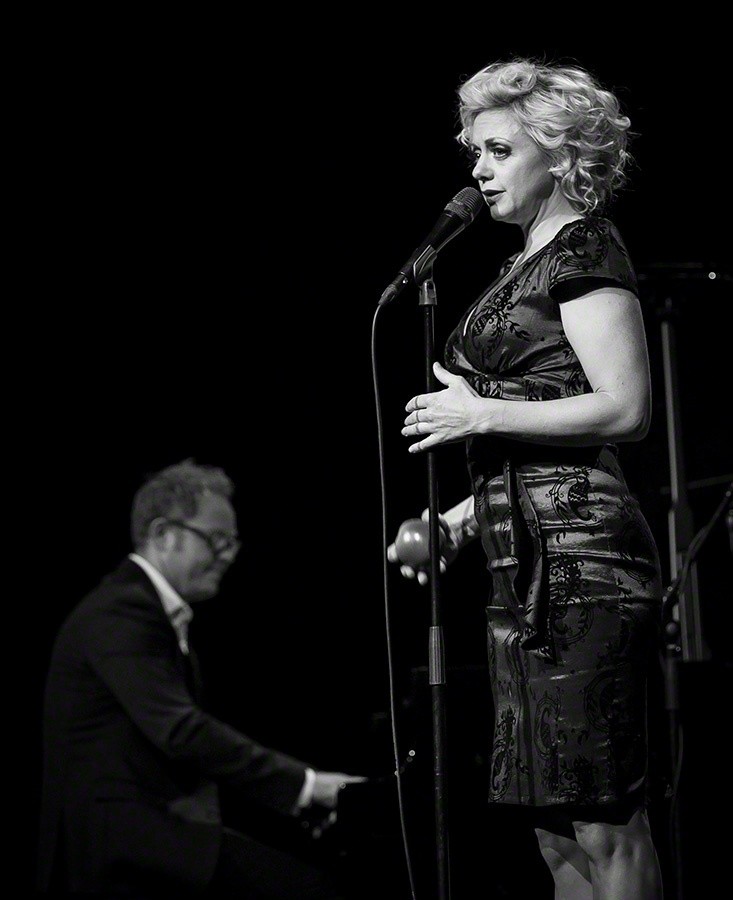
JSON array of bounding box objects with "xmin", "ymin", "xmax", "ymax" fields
[
  {"xmin": 33, "ymin": 467, "xmax": 366, "ymax": 900},
  {"xmin": 389, "ymin": 62, "xmax": 667, "ymax": 900}
]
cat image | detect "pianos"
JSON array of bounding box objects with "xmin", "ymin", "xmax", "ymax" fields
[{"xmin": 299, "ymin": 472, "xmax": 733, "ymax": 900}]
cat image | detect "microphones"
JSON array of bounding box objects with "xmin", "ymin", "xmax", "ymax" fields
[{"xmin": 377, "ymin": 187, "xmax": 484, "ymax": 308}]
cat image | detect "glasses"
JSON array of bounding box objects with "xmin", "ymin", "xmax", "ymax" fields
[{"xmin": 168, "ymin": 518, "xmax": 239, "ymax": 566}]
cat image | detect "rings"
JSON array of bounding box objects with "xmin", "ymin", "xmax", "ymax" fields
[
  {"xmin": 416, "ymin": 422, "xmax": 421, "ymax": 434},
  {"xmin": 416, "ymin": 410, "xmax": 420, "ymax": 422},
  {"xmin": 415, "ymin": 397, "xmax": 420, "ymax": 409}
]
